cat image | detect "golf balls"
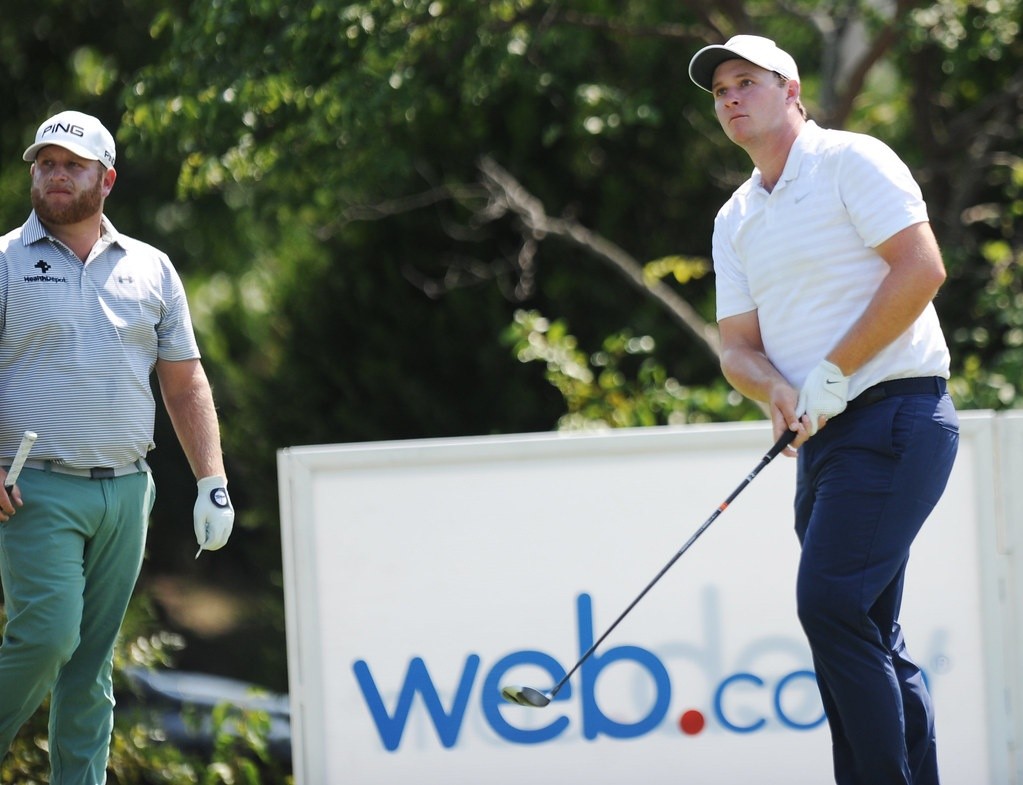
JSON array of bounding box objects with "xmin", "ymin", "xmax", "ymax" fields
[{"xmin": 681, "ymin": 710, "xmax": 704, "ymax": 735}]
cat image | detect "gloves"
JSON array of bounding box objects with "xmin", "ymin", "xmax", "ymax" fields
[
  {"xmin": 795, "ymin": 360, "xmax": 864, "ymax": 440},
  {"xmin": 192, "ymin": 474, "xmax": 233, "ymax": 551}
]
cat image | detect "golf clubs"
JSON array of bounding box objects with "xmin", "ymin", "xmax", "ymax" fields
[
  {"xmin": 501, "ymin": 413, "xmax": 809, "ymax": 707},
  {"xmin": 0, "ymin": 427, "xmax": 39, "ymax": 513}
]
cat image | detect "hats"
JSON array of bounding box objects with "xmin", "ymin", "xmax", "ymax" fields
[
  {"xmin": 688, "ymin": 35, "xmax": 803, "ymax": 86},
  {"xmin": 22, "ymin": 111, "xmax": 116, "ymax": 168}
]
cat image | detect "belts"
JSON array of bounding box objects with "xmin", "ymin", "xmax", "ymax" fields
[
  {"xmin": 1, "ymin": 458, "xmax": 153, "ymax": 480},
  {"xmin": 846, "ymin": 376, "xmax": 947, "ymax": 412}
]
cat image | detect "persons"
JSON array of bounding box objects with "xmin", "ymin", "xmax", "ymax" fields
[
  {"xmin": 688, "ymin": 34, "xmax": 964, "ymax": 785},
  {"xmin": 0, "ymin": 110, "xmax": 234, "ymax": 785}
]
